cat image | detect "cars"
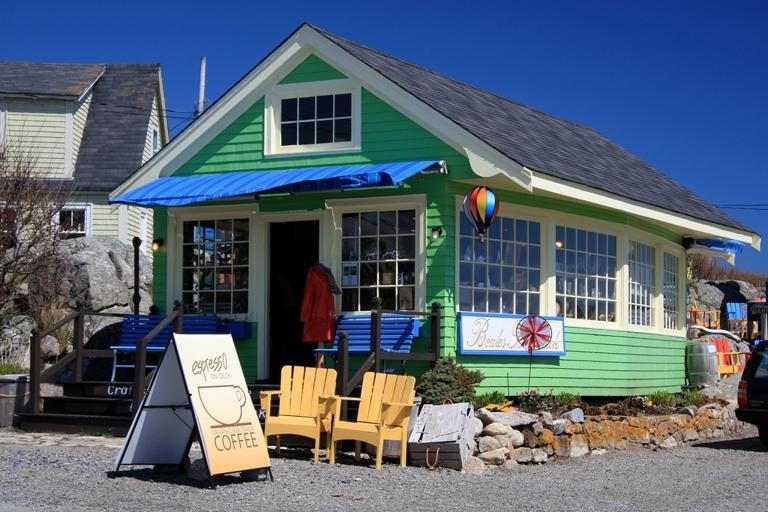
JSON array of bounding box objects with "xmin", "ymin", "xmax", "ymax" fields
[{"xmin": 734, "ymin": 340, "xmax": 768, "ymax": 448}]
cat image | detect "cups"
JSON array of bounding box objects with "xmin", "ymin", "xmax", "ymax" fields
[{"xmin": 198, "ymin": 384, "xmax": 246, "ymax": 425}]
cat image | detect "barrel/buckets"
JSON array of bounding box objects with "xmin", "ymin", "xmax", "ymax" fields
[
  {"xmin": 0, "ymin": 382, "xmax": 32, "ymax": 427},
  {"xmin": 685, "ymin": 342, "xmax": 717, "ymax": 384}
]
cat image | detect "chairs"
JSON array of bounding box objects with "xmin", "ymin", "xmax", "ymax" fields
[{"xmin": 255, "ymin": 363, "xmax": 420, "ymax": 469}]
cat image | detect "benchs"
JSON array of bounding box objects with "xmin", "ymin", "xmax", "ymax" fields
[
  {"xmin": 107, "ymin": 311, "xmax": 219, "ymax": 383},
  {"xmin": 312, "ymin": 312, "xmax": 419, "ymax": 375}
]
